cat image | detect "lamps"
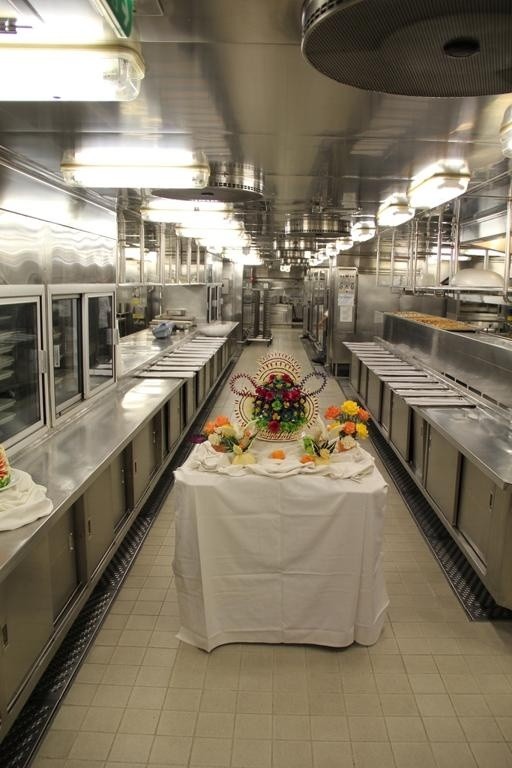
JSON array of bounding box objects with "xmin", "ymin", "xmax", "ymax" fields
[{"xmin": 308, "ymin": 176, "xmax": 472, "ymax": 267}]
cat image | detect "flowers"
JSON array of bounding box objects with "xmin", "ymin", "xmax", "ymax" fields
[
  {"xmin": 251, "ymin": 373, "xmax": 309, "ymax": 433},
  {"xmin": 324, "ymin": 400, "xmax": 369, "ymax": 454},
  {"xmin": 202, "ymin": 416, "xmax": 239, "ymax": 452}
]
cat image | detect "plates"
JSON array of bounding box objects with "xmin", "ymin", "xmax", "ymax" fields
[
  {"xmin": 0, "ymin": 469, "xmax": 23, "ymax": 491},
  {"xmin": 0, "ymin": 328, "xmax": 22, "ymax": 427}
]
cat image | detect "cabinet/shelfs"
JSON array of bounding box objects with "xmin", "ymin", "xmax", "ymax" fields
[{"xmin": 271, "ymin": 304, "xmax": 293, "ymax": 325}]
